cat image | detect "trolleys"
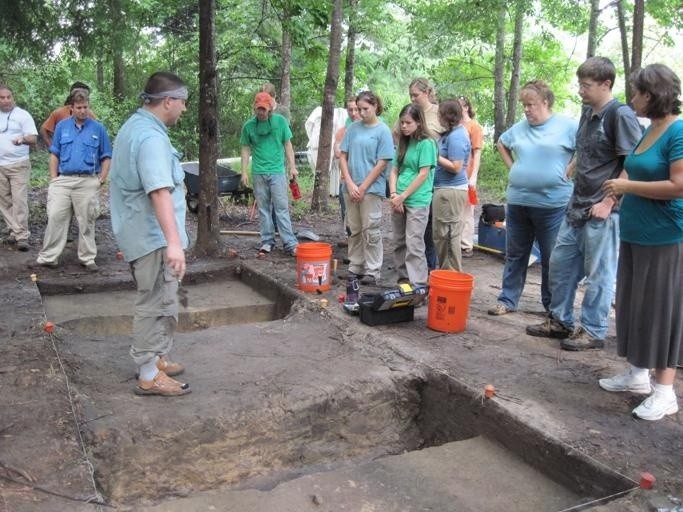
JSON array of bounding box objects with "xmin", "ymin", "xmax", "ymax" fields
[{"xmin": 178, "ymin": 163, "xmax": 254, "ymax": 214}]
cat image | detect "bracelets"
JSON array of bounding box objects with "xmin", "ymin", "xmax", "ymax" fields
[
  {"xmin": 99, "ymin": 176, "xmax": 104, "ymax": 185},
  {"xmin": 609, "ymin": 194, "xmax": 620, "ymax": 207}
]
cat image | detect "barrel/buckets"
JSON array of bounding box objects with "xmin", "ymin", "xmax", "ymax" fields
[
  {"xmin": 295, "ymin": 242, "xmax": 332, "ymax": 292},
  {"xmin": 427, "ymin": 270, "xmax": 474, "ymax": 331}
]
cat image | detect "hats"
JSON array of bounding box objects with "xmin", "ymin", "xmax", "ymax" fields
[{"xmin": 255, "ymin": 93, "xmax": 272, "ymax": 110}]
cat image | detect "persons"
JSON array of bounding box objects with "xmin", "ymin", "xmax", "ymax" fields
[
  {"xmin": 258, "ymin": 83, "xmax": 291, "ymax": 231},
  {"xmin": 39, "ymin": 80, "xmax": 102, "ymax": 153},
  {"xmin": 25, "ymin": 89, "xmax": 111, "ymax": 276},
  {"xmin": 240, "ymin": 91, "xmax": 300, "ymax": 258},
  {"xmin": 107, "ymin": 71, "xmax": 194, "ymax": 395},
  {"xmin": 332, "ymin": 77, "xmax": 482, "ymax": 306},
  {"xmin": 524, "ymin": 48, "xmax": 643, "ymax": 350},
  {"xmin": 487, "ymin": 82, "xmax": 577, "ymax": 315},
  {"xmin": 0, "ymin": 85, "xmax": 37, "ymax": 249},
  {"xmin": 598, "ymin": 60, "xmax": 682, "ymax": 424}
]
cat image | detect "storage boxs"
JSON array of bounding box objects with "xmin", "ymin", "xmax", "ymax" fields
[{"xmin": 353, "ymin": 281, "xmax": 427, "ymax": 326}]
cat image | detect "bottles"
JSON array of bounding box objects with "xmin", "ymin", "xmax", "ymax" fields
[
  {"xmin": 468, "ymin": 184, "xmax": 478, "ymax": 206},
  {"xmin": 288, "ymin": 178, "xmax": 301, "ymax": 200},
  {"xmin": 345, "ymin": 274, "xmax": 359, "ymax": 305}
]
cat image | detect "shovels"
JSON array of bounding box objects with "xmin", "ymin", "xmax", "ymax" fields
[
  {"xmin": 219, "ymin": 229, "xmax": 320, "ymax": 241},
  {"xmin": 473, "ymin": 243, "xmax": 538, "ymax": 268}
]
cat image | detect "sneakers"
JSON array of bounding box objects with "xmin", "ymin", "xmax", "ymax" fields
[
  {"xmin": 137, "ymin": 360, "xmax": 185, "ymax": 376},
  {"xmin": 258, "ymin": 238, "xmax": 375, "ymax": 284},
  {"xmin": 135, "ymin": 370, "xmax": 190, "ymax": 397},
  {"xmin": 527, "ymin": 319, "xmax": 570, "ymax": 337},
  {"xmin": 632, "ymin": 391, "xmax": 679, "ymax": 420},
  {"xmin": 487, "ymin": 303, "xmax": 512, "ymax": 316},
  {"xmin": 562, "ymin": 328, "xmax": 604, "ymax": 350},
  {"xmin": 598, "ymin": 371, "xmax": 651, "ymax": 395},
  {"xmin": 7, "ymin": 236, "xmax": 98, "ymax": 271}
]
